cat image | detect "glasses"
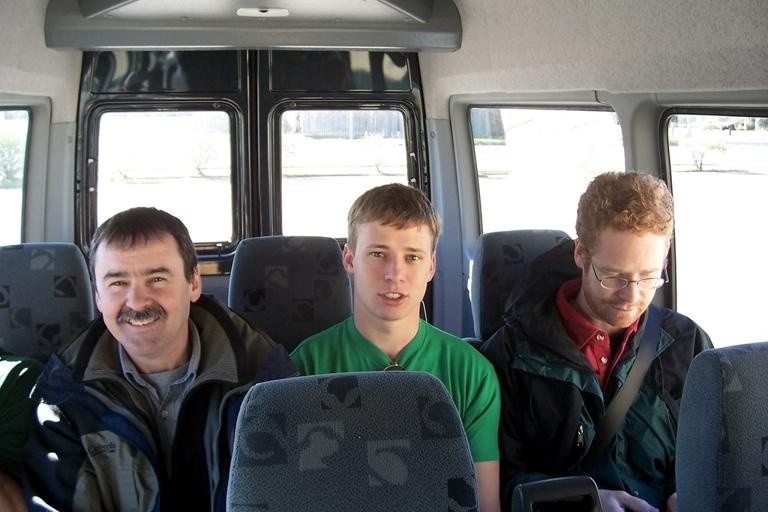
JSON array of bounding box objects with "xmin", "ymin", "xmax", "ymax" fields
[{"xmin": 583, "ymin": 245, "xmax": 671, "ymax": 295}]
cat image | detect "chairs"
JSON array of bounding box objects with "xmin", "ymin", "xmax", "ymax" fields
[
  {"xmin": 0, "ymin": 243, "xmax": 97, "ymax": 360},
  {"xmin": 226, "ymin": 370, "xmax": 479, "ymax": 512},
  {"xmin": 461, "ymin": 229, "xmax": 581, "ymax": 350},
  {"xmin": 228, "ymin": 235, "xmax": 353, "ymax": 354},
  {"xmin": 675, "ymin": 342, "xmax": 767, "ymax": 512}
]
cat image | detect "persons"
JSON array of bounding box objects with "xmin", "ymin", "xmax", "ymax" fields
[
  {"xmin": 0, "ymin": 347, "xmax": 45, "ymax": 512},
  {"xmin": 478, "ymin": 169, "xmax": 715, "ymax": 512},
  {"xmin": 286, "ymin": 182, "xmax": 502, "ymax": 512},
  {"xmin": 16, "ymin": 206, "xmax": 299, "ymax": 511}
]
